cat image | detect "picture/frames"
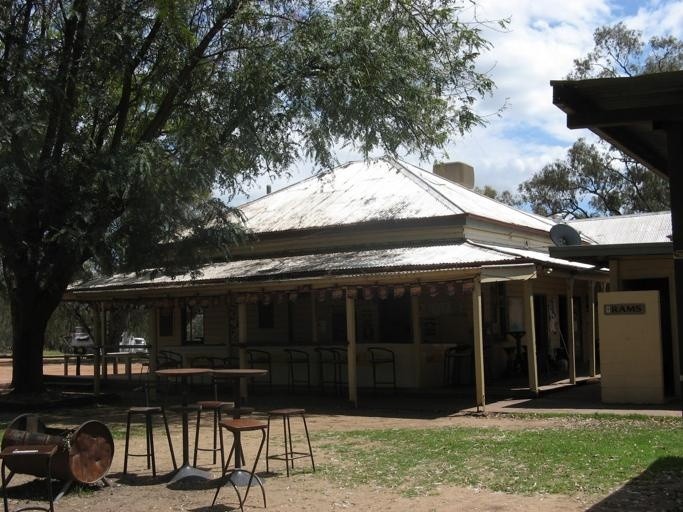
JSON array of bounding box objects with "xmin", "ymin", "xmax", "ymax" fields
[{"xmin": 152, "ymin": 367, "xmax": 268, "ymax": 488}]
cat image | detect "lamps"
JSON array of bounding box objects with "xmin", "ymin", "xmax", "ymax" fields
[
  {"xmin": 444, "ymin": 345, "xmax": 474, "ymax": 387},
  {"xmin": 143, "ymin": 346, "xmax": 397, "ymax": 395}
]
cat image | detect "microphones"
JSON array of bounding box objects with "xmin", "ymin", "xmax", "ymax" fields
[{"xmin": 124, "ymin": 399, "xmax": 315, "ymax": 511}]
